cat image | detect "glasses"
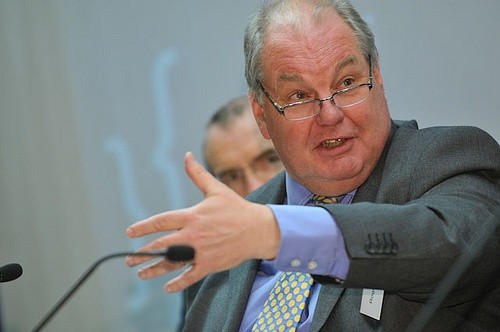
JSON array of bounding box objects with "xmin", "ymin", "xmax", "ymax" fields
[{"xmin": 257, "ymin": 55, "xmax": 374, "ymax": 122}]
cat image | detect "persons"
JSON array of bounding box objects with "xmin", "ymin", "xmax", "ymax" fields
[
  {"xmin": 125, "ymin": 0, "xmax": 500, "ymax": 332},
  {"xmin": 203, "ymin": 95, "xmax": 286, "ymax": 199}
]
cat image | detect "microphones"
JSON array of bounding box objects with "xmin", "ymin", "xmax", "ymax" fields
[
  {"xmin": 0, "ymin": 263, "xmax": 23, "ymax": 282},
  {"xmin": 32, "ymin": 243, "xmax": 195, "ymax": 332}
]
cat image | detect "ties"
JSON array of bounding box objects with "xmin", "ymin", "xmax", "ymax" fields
[{"xmin": 252, "ymin": 193, "xmax": 345, "ymax": 332}]
